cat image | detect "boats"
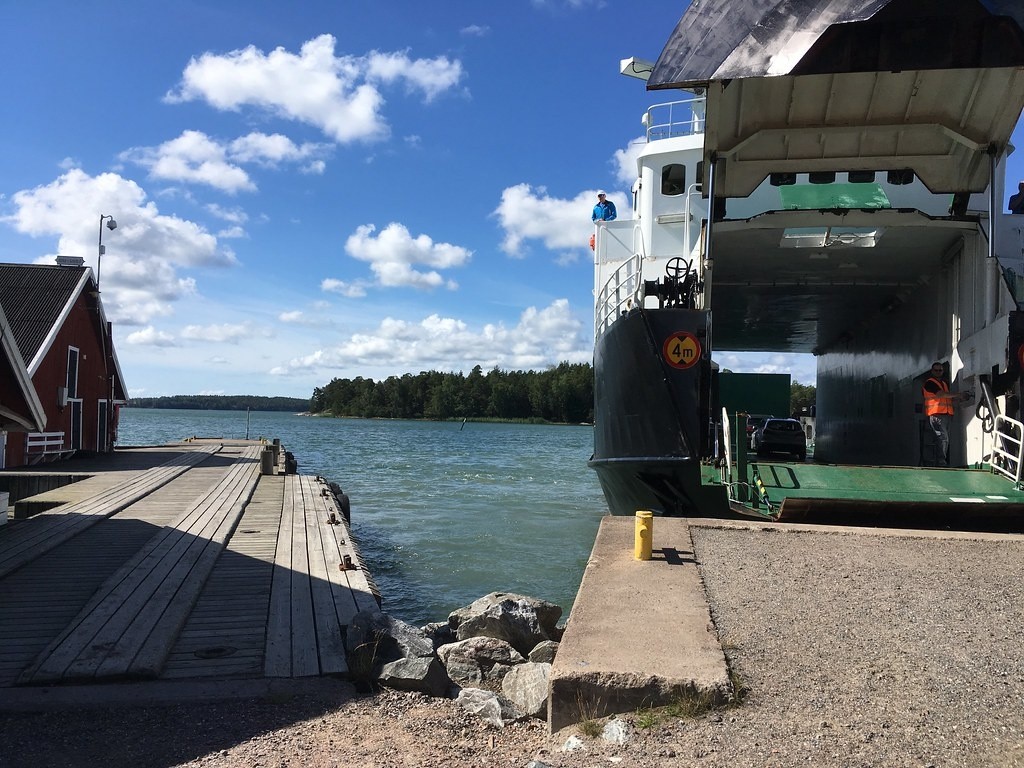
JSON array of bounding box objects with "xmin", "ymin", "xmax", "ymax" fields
[{"xmin": 588, "ymin": 0, "xmax": 1023, "ymax": 520}]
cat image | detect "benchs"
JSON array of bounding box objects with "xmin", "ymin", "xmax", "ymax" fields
[{"xmin": 23, "ymin": 431, "xmax": 77, "ymax": 465}]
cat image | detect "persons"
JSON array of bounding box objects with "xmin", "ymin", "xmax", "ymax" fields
[
  {"xmin": 591, "ymin": 190, "xmax": 617, "ymax": 223},
  {"xmin": 1008, "ymin": 182, "xmax": 1024, "ymax": 214},
  {"xmin": 921, "ymin": 362, "xmax": 969, "ymax": 467}
]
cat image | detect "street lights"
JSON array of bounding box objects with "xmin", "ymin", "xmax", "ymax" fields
[{"xmin": 95, "ymin": 215, "xmax": 117, "ymax": 313}]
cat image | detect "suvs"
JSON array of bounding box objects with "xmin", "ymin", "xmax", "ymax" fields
[
  {"xmin": 751, "ymin": 417, "xmax": 806, "ymax": 460},
  {"xmin": 746, "ymin": 414, "xmax": 774, "ymax": 446}
]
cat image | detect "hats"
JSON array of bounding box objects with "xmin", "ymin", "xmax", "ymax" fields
[{"xmin": 597, "ymin": 190, "xmax": 605, "ymax": 197}]
